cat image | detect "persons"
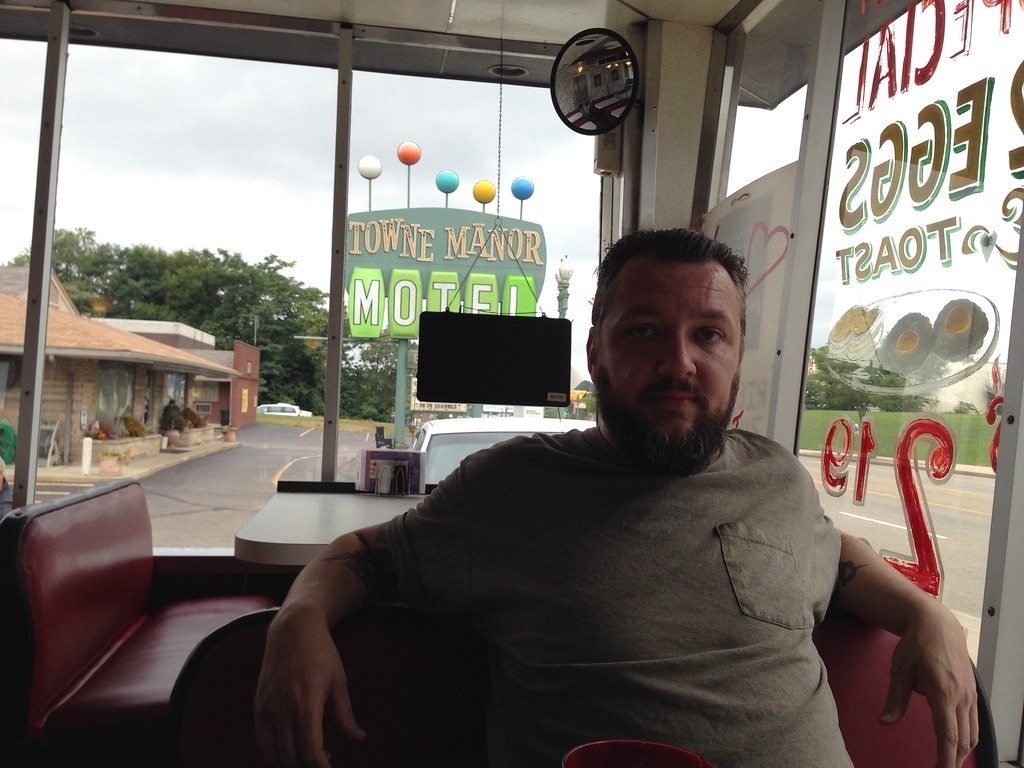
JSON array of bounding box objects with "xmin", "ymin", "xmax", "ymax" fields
[
  {"xmin": 253, "ymin": 228, "xmax": 981, "ymax": 768},
  {"xmin": 0, "ymin": 456, "xmax": 12, "ymax": 522}
]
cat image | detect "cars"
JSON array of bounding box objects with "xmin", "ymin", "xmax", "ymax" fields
[
  {"xmin": 410, "ymin": 416, "xmax": 597, "ymax": 485},
  {"xmin": 257, "ymin": 402, "xmax": 314, "ymax": 418}
]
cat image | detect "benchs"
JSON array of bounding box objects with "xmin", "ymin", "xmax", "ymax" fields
[
  {"xmin": 0, "ymin": 478, "xmax": 274, "ymax": 768},
  {"xmin": 152, "ymin": 607, "xmax": 999, "ymax": 768}
]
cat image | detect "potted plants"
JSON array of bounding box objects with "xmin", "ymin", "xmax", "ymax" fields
[{"xmin": 160, "ymin": 399, "xmax": 187, "ymax": 446}]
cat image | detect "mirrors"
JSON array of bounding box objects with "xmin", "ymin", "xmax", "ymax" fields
[{"xmin": 550, "ymin": 27, "xmax": 640, "ymax": 135}]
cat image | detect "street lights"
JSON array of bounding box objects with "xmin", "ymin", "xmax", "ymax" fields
[{"xmin": 556, "ymin": 254, "xmax": 575, "ymax": 318}]
